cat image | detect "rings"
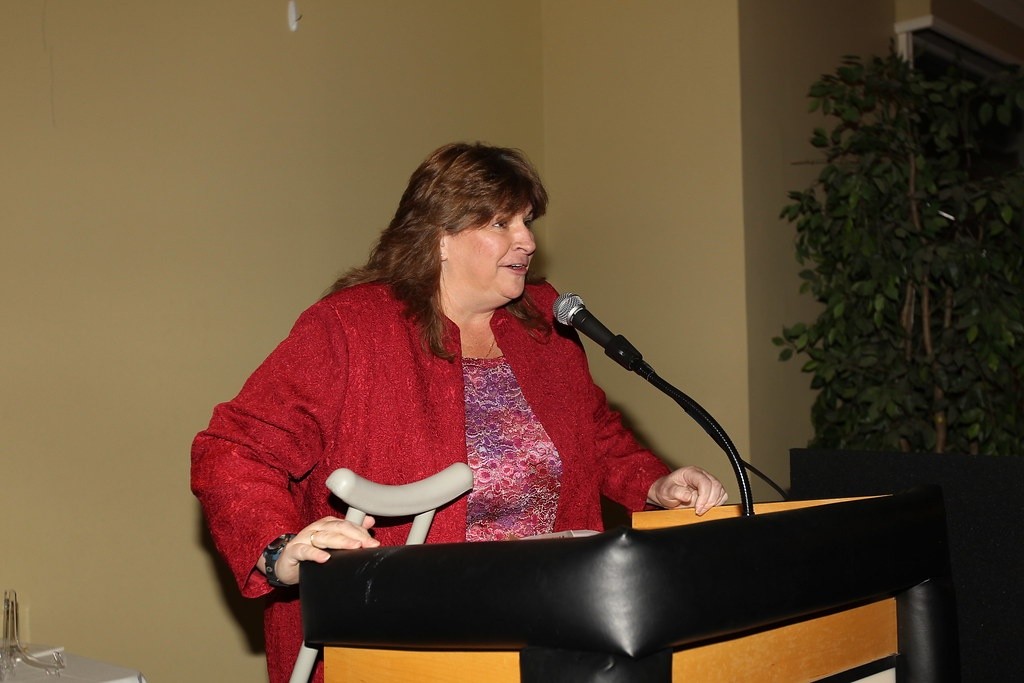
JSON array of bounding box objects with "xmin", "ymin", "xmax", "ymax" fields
[{"xmin": 310, "ymin": 531, "xmax": 320, "ymax": 548}]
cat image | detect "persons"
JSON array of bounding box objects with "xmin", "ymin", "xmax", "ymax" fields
[{"xmin": 191, "ymin": 142, "xmax": 729, "ymax": 683}]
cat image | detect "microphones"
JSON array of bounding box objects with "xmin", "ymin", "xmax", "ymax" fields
[{"xmin": 553, "ymin": 291, "xmax": 644, "ymax": 370}]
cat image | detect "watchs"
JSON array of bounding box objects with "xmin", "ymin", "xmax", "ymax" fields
[{"xmin": 263, "ymin": 534, "xmax": 296, "ymax": 589}]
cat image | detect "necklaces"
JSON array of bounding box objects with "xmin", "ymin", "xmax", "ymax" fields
[{"xmin": 486, "ymin": 341, "xmax": 495, "ymax": 357}]
